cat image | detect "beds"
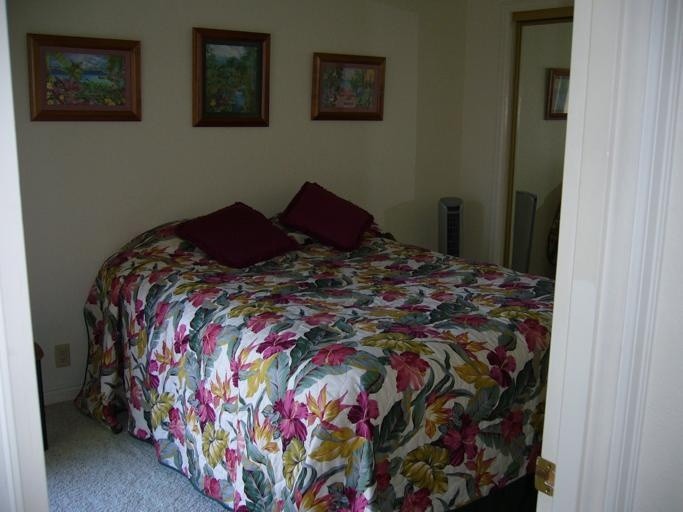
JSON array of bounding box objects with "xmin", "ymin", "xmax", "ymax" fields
[{"xmin": 72, "ymin": 208, "xmax": 555, "ymax": 512}]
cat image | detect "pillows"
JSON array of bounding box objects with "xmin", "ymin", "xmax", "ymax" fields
[{"xmin": 175, "ymin": 182, "xmax": 375, "ymax": 268}]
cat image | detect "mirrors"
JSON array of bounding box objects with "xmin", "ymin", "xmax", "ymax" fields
[{"xmin": 502, "ymin": 5, "xmax": 573, "ymax": 280}]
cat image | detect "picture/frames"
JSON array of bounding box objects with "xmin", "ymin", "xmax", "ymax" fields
[
  {"xmin": 192, "ymin": 27, "xmax": 270, "ymax": 127},
  {"xmin": 26, "ymin": 32, "xmax": 142, "ymax": 122},
  {"xmin": 310, "ymin": 51, "xmax": 386, "ymax": 121},
  {"xmin": 545, "ymin": 67, "xmax": 570, "ymax": 120}
]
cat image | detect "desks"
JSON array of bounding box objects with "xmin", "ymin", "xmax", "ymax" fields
[{"xmin": 33, "ymin": 340, "xmax": 49, "ymax": 452}]
